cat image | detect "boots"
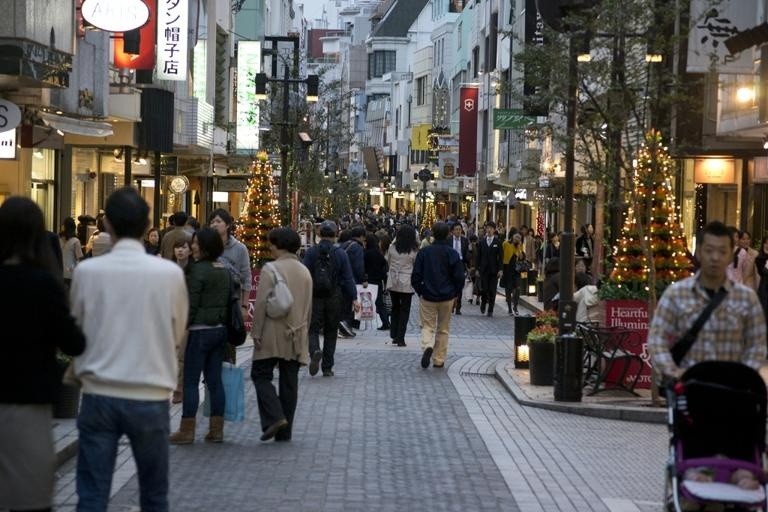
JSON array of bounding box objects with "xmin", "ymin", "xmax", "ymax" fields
[
  {"xmin": 169, "ymin": 418, "xmax": 195, "ymax": 445},
  {"xmin": 204, "ymin": 415, "xmax": 224, "ymax": 443}
]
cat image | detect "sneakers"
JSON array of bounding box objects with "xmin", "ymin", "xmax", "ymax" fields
[
  {"xmin": 258, "ymin": 417, "xmax": 290, "ymax": 443},
  {"xmin": 308, "ymin": 298, "xmax": 520, "ymax": 378}
]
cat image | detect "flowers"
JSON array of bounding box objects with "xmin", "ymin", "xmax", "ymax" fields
[{"xmin": 528, "ymin": 310, "xmax": 559, "ymax": 341}]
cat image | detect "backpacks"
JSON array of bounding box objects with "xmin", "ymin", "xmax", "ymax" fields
[{"xmin": 312, "ymin": 243, "xmax": 341, "ymax": 297}]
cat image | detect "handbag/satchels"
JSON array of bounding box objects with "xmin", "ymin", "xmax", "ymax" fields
[
  {"xmin": 451, "ymin": 262, "xmax": 467, "ymax": 288},
  {"xmin": 202, "ymin": 364, "xmax": 246, "ymax": 422},
  {"xmin": 514, "ymin": 260, "xmax": 532, "ymax": 272},
  {"xmin": 228, "ymin": 295, "xmax": 246, "ymax": 343},
  {"xmin": 366, "ymin": 248, "xmax": 386, "ymax": 267},
  {"xmin": 267, "ymin": 282, "xmax": 294, "ymax": 319},
  {"xmin": 351, "ymin": 284, "xmax": 379, "ymax": 320}
]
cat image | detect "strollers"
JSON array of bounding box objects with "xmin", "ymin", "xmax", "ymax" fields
[{"xmin": 661, "ymin": 359, "xmax": 768, "ymax": 511}]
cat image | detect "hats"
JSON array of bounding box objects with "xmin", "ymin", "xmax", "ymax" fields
[
  {"xmin": 319, "ymin": 219, "xmax": 341, "ymax": 237},
  {"xmin": 485, "ymin": 222, "xmax": 497, "ymax": 227}
]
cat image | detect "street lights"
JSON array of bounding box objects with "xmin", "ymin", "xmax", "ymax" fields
[{"xmin": 251, "ymin": 72, "xmax": 322, "ymax": 256}]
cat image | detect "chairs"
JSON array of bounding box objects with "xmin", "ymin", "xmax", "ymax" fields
[{"xmin": 576, "ymin": 319, "xmax": 643, "ymax": 396}]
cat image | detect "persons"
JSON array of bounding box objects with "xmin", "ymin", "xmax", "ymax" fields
[
  {"xmin": 359, "ymin": 204, "xmax": 562, "ymax": 317},
  {"xmin": 684, "ymin": 450, "xmax": 761, "ymax": 491},
  {"xmin": 67, "ymin": 184, "xmax": 190, "ymax": 512},
  {"xmin": 725, "ymin": 225, "xmax": 747, "ymax": 286},
  {"xmin": 384, "ymin": 224, "xmax": 419, "ymax": 346},
  {"xmin": 574, "ymin": 222, "xmax": 600, "ymax": 327},
  {"xmin": 647, "ymin": 214, "xmax": 767, "ymax": 402},
  {"xmin": 755, "ymin": 233, "xmax": 767, "ymax": 322},
  {"xmin": 303, "ymin": 217, "xmax": 388, "ymax": 376},
  {"xmin": 250, "ymin": 229, "xmax": 314, "ymax": 448},
  {"xmin": 0, "ymin": 195, "xmax": 85, "ymax": 512},
  {"xmin": 739, "ymin": 231, "xmax": 761, "ymax": 293},
  {"xmin": 48, "ymin": 205, "xmax": 253, "ymax": 446},
  {"xmin": 412, "ymin": 222, "xmax": 466, "ymax": 368}
]
